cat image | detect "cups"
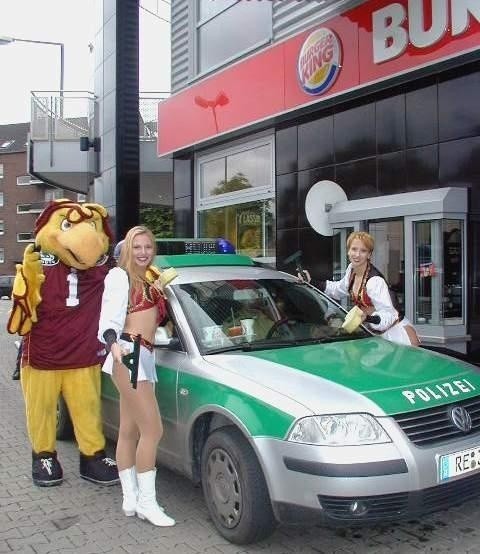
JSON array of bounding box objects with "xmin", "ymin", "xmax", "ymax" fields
[
  {"xmin": 228, "ymin": 325, "xmax": 242, "ymax": 337},
  {"xmin": 202, "ymin": 326, "xmax": 213, "ymax": 340},
  {"xmin": 213, "ymin": 324, "xmax": 224, "ymax": 340},
  {"xmin": 240, "ymin": 318, "xmax": 255, "ymax": 335}
]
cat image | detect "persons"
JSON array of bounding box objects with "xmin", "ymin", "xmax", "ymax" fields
[
  {"xmin": 221, "ymin": 287, "xmax": 280, "ymax": 345},
  {"xmin": 94, "ymin": 226, "xmax": 178, "ymax": 529},
  {"xmin": 291, "ymin": 228, "xmax": 423, "ymax": 349}
]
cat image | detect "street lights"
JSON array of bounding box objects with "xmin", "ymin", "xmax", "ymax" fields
[{"xmin": 1, "ymin": 34, "xmax": 69, "ymax": 127}]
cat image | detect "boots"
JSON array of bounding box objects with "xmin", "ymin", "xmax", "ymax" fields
[
  {"xmin": 137, "ymin": 467, "xmax": 176, "ymax": 527},
  {"xmin": 118, "ymin": 465, "xmax": 164, "ymax": 516}
]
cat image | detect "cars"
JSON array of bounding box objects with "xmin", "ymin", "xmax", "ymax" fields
[
  {"xmin": 0, "ymin": 275, "xmax": 16, "ymax": 302},
  {"xmin": 40, "ymin": 237, "xmax": 480, "ymax": 542}
]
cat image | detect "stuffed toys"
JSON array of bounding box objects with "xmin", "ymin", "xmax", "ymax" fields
[{"xmin": 6, "ymin": 196, "xmax": 127, "ymax": 485}]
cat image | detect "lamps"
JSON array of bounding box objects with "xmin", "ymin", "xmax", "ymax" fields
[{"xmin": 80, "ymin": 137, "xmax": 101, "ymax": 151}]
